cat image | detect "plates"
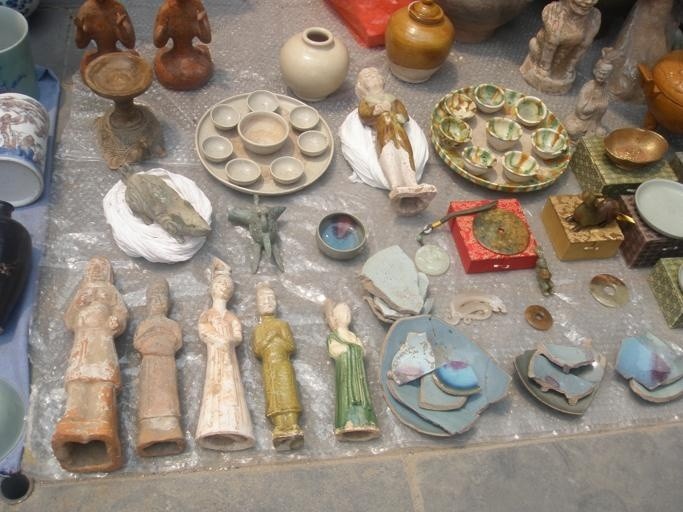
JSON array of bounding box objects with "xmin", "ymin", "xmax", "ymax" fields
[
  {"xmin": 193, "ymin": 90, "xmax": 336, "ymax": 197},
  {"xmin": 429, "ymin": 84, "xmax": 571, "ymax": 193},
  {"xmin": 633, "ymin": 176, "xmax": 683, "ymax": 240}
]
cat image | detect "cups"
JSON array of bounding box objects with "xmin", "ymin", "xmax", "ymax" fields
[
  {"xmin": 0, "ymin": 4, "xmax": 36, "ymax": 104},
  {"xmin": 200, "ymin": 90, "xmax": 331, "ymax": 188},
  {"xmin": 440, "ymin": 84, "xmax": 568, "ymax": 181},
  {"xmin": 0, "ymin": 90, "xmax": 51, "ymax": 207}
]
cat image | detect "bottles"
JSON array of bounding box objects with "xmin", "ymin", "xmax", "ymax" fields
[{"xmin": 0, "ymin": 199, "xmax": 34, "ymax": 339}]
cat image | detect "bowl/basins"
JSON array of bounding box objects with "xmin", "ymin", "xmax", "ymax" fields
[
  {"xmin": 314, "ymin": 211, "xmax": 368, "ymax": 260},
  {"xmin": 600, "ymin": 126, "xmax": 670, "ymax": 171},
  {"xmin": 0, "ymin": 378, "xmax": 30, "ymax": 462}
]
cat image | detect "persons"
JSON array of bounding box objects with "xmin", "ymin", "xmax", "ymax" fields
[
  {"xmin": 519, "ymin": 1, "xmax": 602, "ymax": 94},
  {"xmin": 48, "ymin": 256, "xmax": 129, "ymax": 473},
  {"xmin": 565, "ymin": 61, "xmax": 613, "ymax": 141},
  {"xmin": 251, "ymin": 286, "xmax": 306, "ymax": 452},
  {"xmin": 192, "ymin": 258, "xmax": 256, "ymax": 451},
  {"xmin": 355, "ymin": 66, "xmax": 438, "ymax": 218},
  {"xmin": 132, "ymin": 276, "xmax": 190, "ymax": 458},
  {"xmin": 325, "ymin": 302, "xmax": 381, "ymax": 442}
]
